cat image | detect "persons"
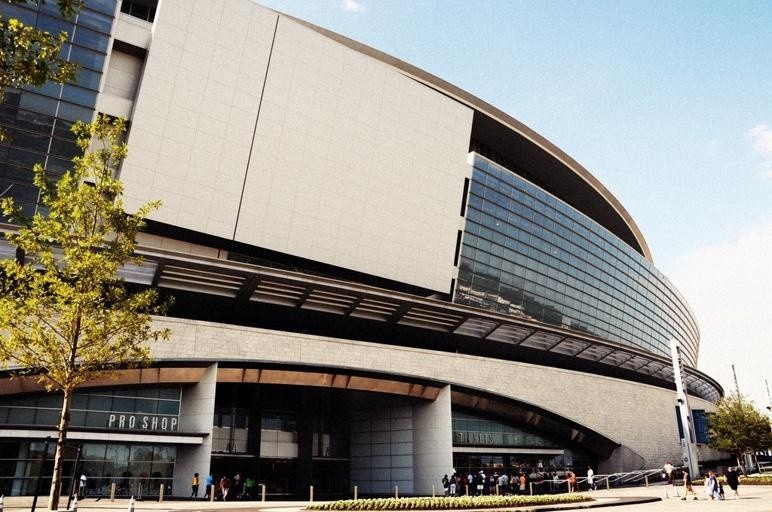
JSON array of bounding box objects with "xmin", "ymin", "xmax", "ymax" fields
[
  {"xmin": 679, "ymin": 467, "xmax": 698, "ymax": 500},
  {"xmin": 662, "ymin": 460, "xmax": 677, "ymax": 485},
  {"xmin": 707, "ymin": 470, "xmax": 721, "ymax": 501},
  {"xmin": 704, "ymin": 473, "xmax": 711, "ymax": 499},
  {"xmin": 190, "ymin": 472, "xmax": 200, "ymax": 501},
  {"xmin": 585, "ymin": 464, "xmax": 598, "ymax": 490},
  {"xmin": 437, "ymin": 456, "xmax": 579, "ymax": 495},
  {"xmin": 203, "ymin": 471, "xmax": 216, "ymax": 501},
  {"xmin": 215, "ymin": 471, "xmax": 257, "ymax": 501},
  {"xmin": 715, "ymin": 478, "xmax": 725, "ymax": 499},
  {"xmin": 724, "ymin": 466, "xmax": 741, "ymax": 500}
]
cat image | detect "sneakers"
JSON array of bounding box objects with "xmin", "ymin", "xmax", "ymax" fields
[
  {"xmin": 663, "ymin": 494, "xmax": 681, "ymax": 499},
  {"xmin": 681, "ymin": 497, "xmax": 698, "ymax": 501},
  {"xmin": 588, "ymin": 486, "xmax": 598, "ymax": 491},
  {"xmin": 732, "ymin": 495, "xmax": 741, "ymax": 500},
  {"xmin": 708, "ymin": 496, "xmax": 726, "ymax": 501}
]
